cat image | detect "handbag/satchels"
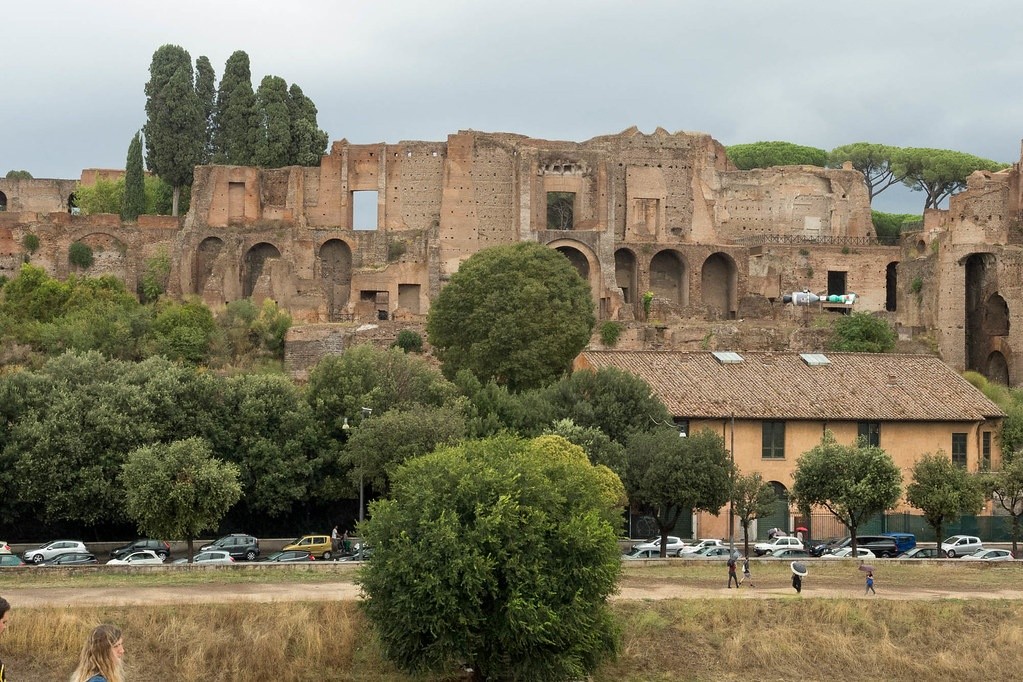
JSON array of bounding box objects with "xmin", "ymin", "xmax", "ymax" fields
[{"xmin": 868, "ymin": 580, "xmax": 872, "ymax": 587}]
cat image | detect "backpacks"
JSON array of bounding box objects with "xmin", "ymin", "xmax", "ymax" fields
[{"xmin": 741, "ymin": 564, "xmax": 746, "ymax": 572}]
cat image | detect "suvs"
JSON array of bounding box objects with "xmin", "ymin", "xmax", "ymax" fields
[
  {"xmin": 23, "ymin": 539, "xmax": 91, "ymax": 561},
  {"xmin": 199, "ymin": 533, "xmax": 260, "ymax": 559},
  {"xmin": 822, "ymin": 548, "xmax": 875, "ymax": 559},
  {"xmin": 36, "ymin": 553, "xmax": 100, "ymax": 564}
]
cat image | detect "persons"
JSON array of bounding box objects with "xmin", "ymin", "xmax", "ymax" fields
[
  {"xmin": 0, "ymin": 597, "xmax": 12, "ymax": 682},
  {"xmin": 798, "ymin": 531, "xmax": 804, "ymax": 542},
  {"xmin": 332, "ymin": 525, "xmax": 350, "ymax": 553},
  {"xmin": 791, "ymin": 573, "xmax": 802, "ymax": 593},
  {"xmin": 727, "ymin": 557, "xmax": 755, "ymax": 588},
  {"xmin": 769, "ymin": 533, "xmax": 773, "ymax": 541},
  {"xmin": 70, "ymin": 624, "xmax": 125, "ymax": 682},
  {"xmin": 864, "ymin": 571, "xmax": 876, "ymax": 595}
]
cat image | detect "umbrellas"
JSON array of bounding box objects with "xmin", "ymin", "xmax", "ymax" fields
[
  {"xmin": 795, "ymin": 527, "xmax": 808, "ymax": 532},
  {"xmin": 859, "ymin": 565, "xmax": 876, "ymax": 571},
  {"xmin": 790, "ymin": 561, "xmax": 809, "ymax": 576}
]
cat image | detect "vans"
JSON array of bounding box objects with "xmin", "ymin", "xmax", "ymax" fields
[
  {"xmin": 822, "ymin": 536, "xmax": 899, "ymax": 558},
  {"xmin": 879, "ymin": 533, "xmax": 917, "ymax": 552}
]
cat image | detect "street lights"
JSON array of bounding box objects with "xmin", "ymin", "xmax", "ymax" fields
[{"xmin": 342, "ymin": 418, "xmax": 363, "ymax": 562}]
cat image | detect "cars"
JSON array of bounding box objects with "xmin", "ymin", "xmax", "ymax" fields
[
  {"xmin": 757, "ymin": 549, "xmax": 808, "ymax": 557},
  {"xmin": 173, "ymin": 550, "xmax": 236, "ymax": 563},
  {"xmin": 752, "ymin": 536, "xmax": 804, "ymax": 554},
  {"xmin": 281, "ymin": 534, "xmax": 333, "ymax": 558},
  {"xmin": 330, "ymin": 547, "xmax": 374, "ymax": 561},
  {"xmin": 621, "ymin": 536, "xmax": 741, "ymax": 559},
  {"xmin": 251, "ymin": 551, "xmax": 314, "ymax": 561},
  {"xmin": 110, "ymin": 538, "xmax": 171, "ymax": 562},
  {"xmin": 107, "ymin": 549, "xmax": 163, "ymax": 564},
  {"xmin": 941, "ymin": 534, "xmax": 983, "ymax": 557},
  {"xmin": 897, "ymin": 546, "xmax": 949, "ymax": 558},
  {"xmin": 810, "ymin": 540, "xmax": 837, "ymax": 557},
  {"xmin": 963, "ymin": 548, "xmax": 1014, "ymax": 561},
  {"xmin": 0, "ymin": 540, "xmax": 26, "ymax": 566}
]
